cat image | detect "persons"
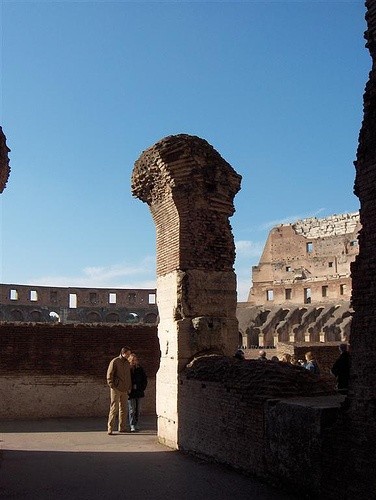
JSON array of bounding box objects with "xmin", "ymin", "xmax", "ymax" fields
[
  {"xmin": 234, "ymin": 349, "xmax": 322, "ymax": 376},
  {"xmin": 330, "ymin": 343, "xmax": 351, "ymax": 395},
  {"xmin": 105, "ymin": 346, "xmax": 133, "ymax": 435},
  {"xmin": 125, "ymin": 353, "xmax": 149, "ymax": 432}
]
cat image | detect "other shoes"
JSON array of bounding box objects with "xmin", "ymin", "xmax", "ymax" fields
[
  {"xmin": 135, "ymin": 426, "xmax": 139, "ymax": 430},
  {"xmin": 121, "ymin": 428, "xmax": 127, "ymax": 432},
  {"xmin": 130, "ymin": 425, "xmax": 136, "ymax": 431},
  {"xmin": 107, "ymin": 430, "xmax": 112, "ymax": 434}
]
